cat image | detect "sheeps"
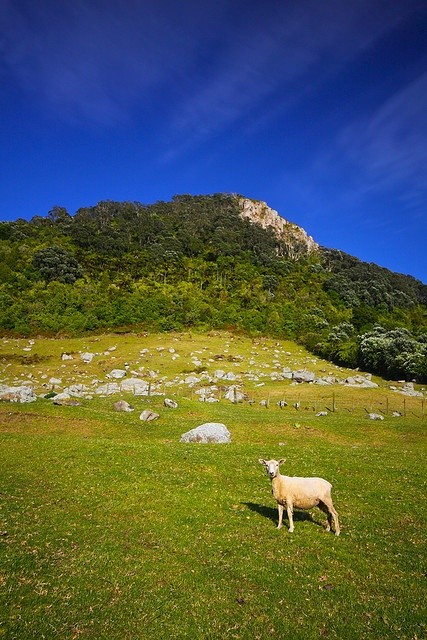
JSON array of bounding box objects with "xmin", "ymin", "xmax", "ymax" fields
[{"xmin": 257, "ymin": 458, "xmax": 341, "ymax": 538}]
